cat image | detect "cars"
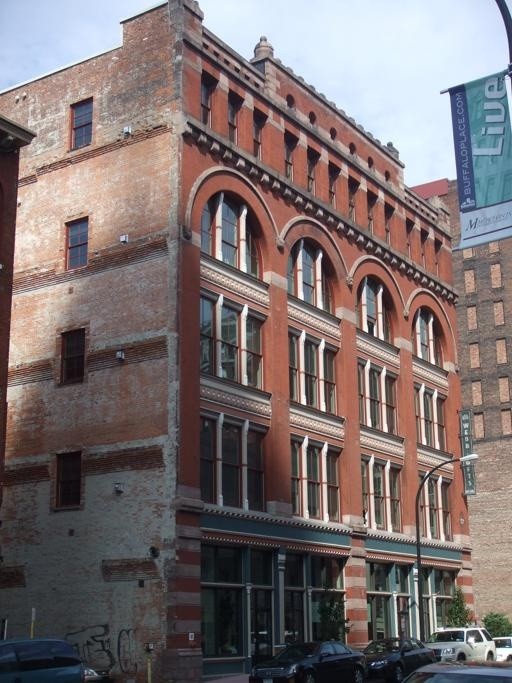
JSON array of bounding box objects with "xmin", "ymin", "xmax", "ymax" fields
[
  {"xmin": 491, "ymin": 637, "xmax": 512, "ymax": 663},
  {"xmin": 397, "ymin": 662, "xmax": 512, "ymax": 683},
  {"xmin": 242, "ymin": 628, "xmax": 385, "ymax": 648},
  {"xmin": 84, "ymin": 661, "xmax": 114, "ymax": 683},
  {"xmin": 364, "ymin": 637, "xmax": 436, "ymax": 683},
  {"xmin": 249, "ymin": 639, "xmax": 366, "ymax": 682}
]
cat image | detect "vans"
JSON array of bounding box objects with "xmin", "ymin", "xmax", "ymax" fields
[{"xmin": 0, "ymin": 637, "xmax": 84, "ymax": 683}]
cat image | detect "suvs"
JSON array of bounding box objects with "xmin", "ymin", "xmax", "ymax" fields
[{"xmin": 421, "ymin": 626, "xmax": 496, "ymax": 664}]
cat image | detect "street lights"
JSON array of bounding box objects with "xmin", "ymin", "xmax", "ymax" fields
[{"xmin": 416, "ymin": 452, "xmax": 479, "ymax": 643}]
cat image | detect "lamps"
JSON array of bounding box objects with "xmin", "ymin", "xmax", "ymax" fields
[
  {"xmin": 123, "ymin": 124, "xmax": 131, "ymax": 135},
  {"xmin": 114, "ymin": 349, "xmax": 125, "ymax": 361},
  {"xmin": 119, "ymin": 233, "xmax": 128, "ymax": 246},
  {"xmin": 112, "ymin": 481, "xmax": 124, "ymax": 492}
]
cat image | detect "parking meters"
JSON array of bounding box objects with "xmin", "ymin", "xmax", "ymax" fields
[{"xmin": 145, "ymin": 641, "xmax": 154, "ymax": 681}]
